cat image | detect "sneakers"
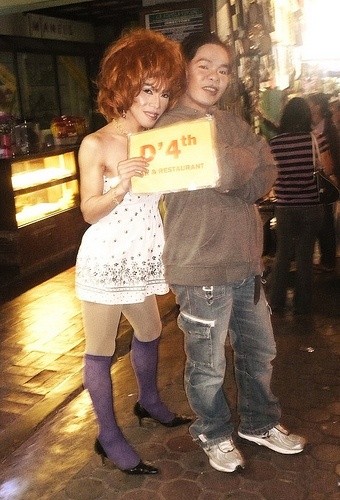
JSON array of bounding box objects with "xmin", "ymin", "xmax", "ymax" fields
[
  {"xmin": 237, "ymin": 421, "xmax": 308, "ymax": 455},
  {"xmin": 192, "ymin": 430, "xmax": 246, "ymax": 473}
]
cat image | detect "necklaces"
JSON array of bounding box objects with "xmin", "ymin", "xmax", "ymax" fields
[{"xmin": 111, "ymin": 119, "xmax": 147, "ymax": 140}]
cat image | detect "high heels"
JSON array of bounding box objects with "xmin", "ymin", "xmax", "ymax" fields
[
  {"xmin": 93, "ymin": 438, "xmax": 159, "ymax": 475},
  {"xmin": 133, "ymin": 401, "xmax": 193, "ymax": 428}
]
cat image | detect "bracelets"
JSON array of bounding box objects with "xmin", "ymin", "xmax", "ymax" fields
[{"xmin": 108, "ymin": 189, "xmax": 121, "ymax": 207}]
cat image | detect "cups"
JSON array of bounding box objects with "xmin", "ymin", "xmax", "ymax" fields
[{"xmin": 0, "ymin": 119, "xmax": 41, "ymax": 159}]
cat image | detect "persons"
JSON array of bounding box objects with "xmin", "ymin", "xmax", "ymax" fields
[
  {"xmin": 72, "ymin": 27, "xmax": 194, "ymax": 475},
  {"xmin": 258, "ymin": 90, "xmax": 340, "ymax": 319},
  {"xmin": 150, "ymin": 31, "xmax": 308, "ymax": 472}
]
